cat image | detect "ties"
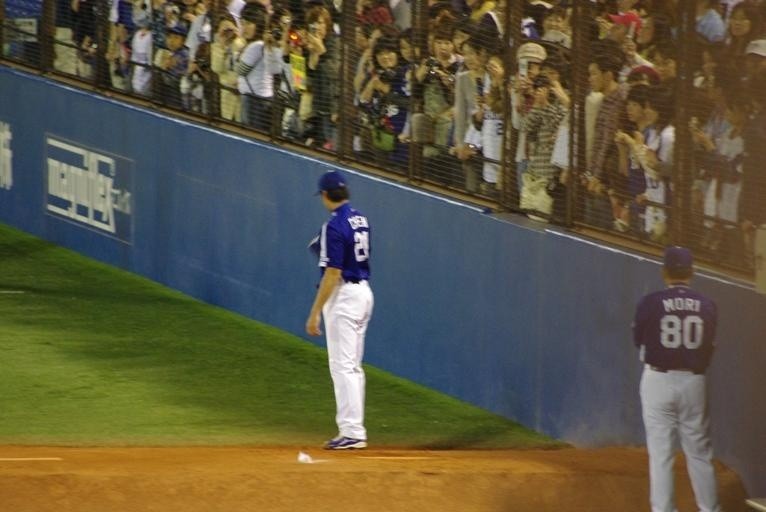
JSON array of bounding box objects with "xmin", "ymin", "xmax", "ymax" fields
[{"xmin": 475, "ymin": 79, "xmax": 484, "ymax": 97}]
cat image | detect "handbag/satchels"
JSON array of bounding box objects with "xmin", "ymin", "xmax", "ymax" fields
[{"xmin": 519, "ymin": 173, "xmax": 554, "ymax": 223}]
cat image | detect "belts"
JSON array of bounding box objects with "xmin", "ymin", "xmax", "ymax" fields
[{"xmin": 652, "ymin": 366, "xmax": 706, "ymax": 375}]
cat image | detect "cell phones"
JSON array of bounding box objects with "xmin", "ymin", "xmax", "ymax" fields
[{"xmin": 625, "ymin": 21, "xmax": 637, "ymax": 41}]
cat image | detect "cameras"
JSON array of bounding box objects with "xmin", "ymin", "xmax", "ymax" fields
[
  {"xmin": 532, "ymin": 75, "xmax": 550, "ymax": 88},
  {"xmin": 426, "ymin": 56, "xmax": 440, "ymax": 67},
  {"xmin": 272, "ymin": 29, "xmax": 282, "ymax": 40}
]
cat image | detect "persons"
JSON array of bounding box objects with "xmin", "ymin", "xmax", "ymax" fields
[
  {"xmin": 43, "ymin": 1, "xmax": 765, "ymax": 289},
  {"xmin": 303, "ymin": 166, "xmax": 376, "ymax": 451},
  {"xmin": 632, "ymin": 245, "xmax": 723, "ymax": 512}
]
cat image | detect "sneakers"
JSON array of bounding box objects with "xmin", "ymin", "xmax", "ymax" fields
[{"xmin": 323, "ymin": 436, "xmax": 367, "ymax": 450}]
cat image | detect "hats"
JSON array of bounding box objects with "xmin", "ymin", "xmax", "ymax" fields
[
  {"xmin": 165, "ymin": 26, "xmax": 189, "ymax": 36},
  {"xmin": 745, "ymin": 39, "xmax": 765, "ymax": 56},
  {"xmin": 609, "ymin": 13, "xmax": 641, "ymax": 30},
  {"xmin": 313, "ymin": 172, "xmax": 347, "ymax": 196},
  {"xmin": 631, "ymin": 65, "xmax": 659, "ymax": 83},
  {"xmin": 516, "ymin": 29, "xmax": 572, "ymax": 64},
  {"xmin": 663, "ymin": 248, "xmax": 692, "ymax": 270}
]
